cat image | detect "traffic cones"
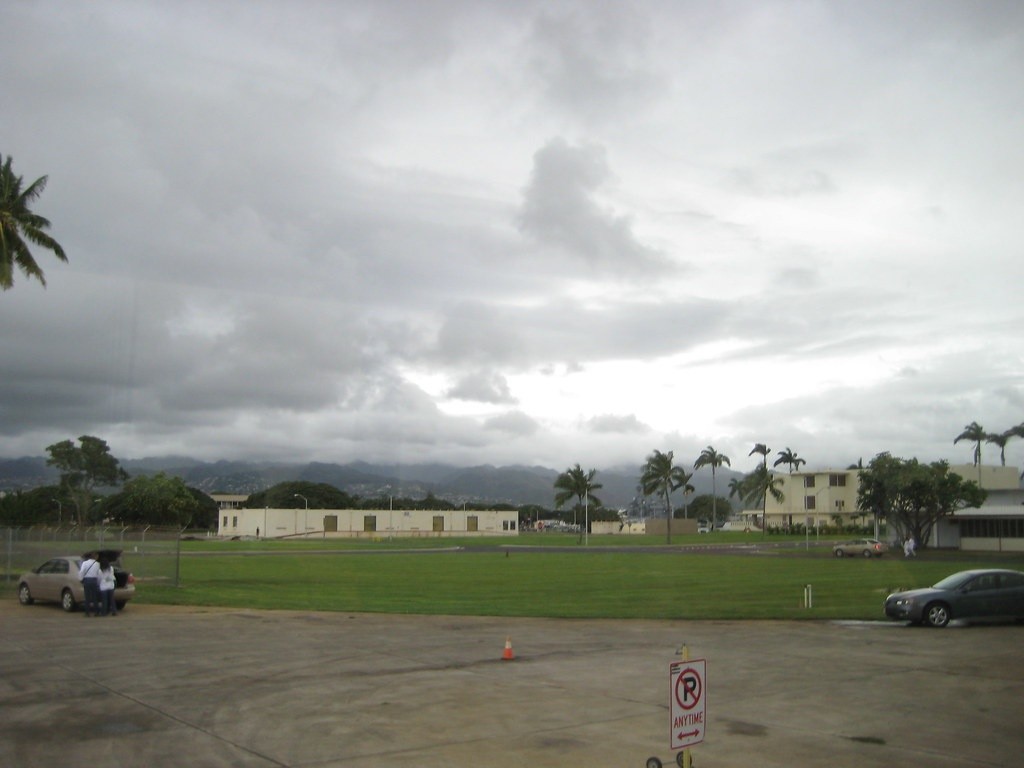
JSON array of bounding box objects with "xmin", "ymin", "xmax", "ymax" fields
[{"xmin": 500, "ymin": 633, "xmax": 519, "ymax": 661}]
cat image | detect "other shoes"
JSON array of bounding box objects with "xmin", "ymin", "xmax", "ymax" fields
[
  {"xmin": 94, "ymin": 611, "xmax": 99, "ymax": 616},
  {"xmin": 112, "ymin": 612, "xmax": 118, "ymax": 616},
  {"xmin": 85, "ymin": 612, "xmax": 90, "ymax": 617}
]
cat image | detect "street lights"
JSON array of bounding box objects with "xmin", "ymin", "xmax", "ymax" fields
[
  {"xmin": 462, "ymin": 499, "xmax": 467, "ymax": 536},
  {"xmin": 51, "ymin": 499, "xmax": 62, "ymax": 527},
  {"xmin": 789, "ymin": 468, "xmax": 810, "ymax": 551},
  {"xmin": 585, "ymin": 480, "xmax": 590, "ymax": 546},
  {"xmin": 816, "ymin": 485, "xmax": 832, "ymax": 541},
  {"xmin": 294, "ymin": 493, "xmax": 308, "ymax": 539},
  {"xmin": 387, "ymin": 493, "xmax": 393, "ymax": 540},
  {"xmin": 263, "ymin": 505, "xmax": 268, "ymax": 536},
  {"xmin": 829, "ymin": 467, "xmax": 841, "ymax": 537}
]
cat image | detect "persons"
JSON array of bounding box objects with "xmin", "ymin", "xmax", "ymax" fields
[
  {"xmin": 903, "ymin": 536, "xmax": 916, "ymax": 557},
  {"xmin": 78, "ymin": 552, "xmax": 118, "ymax": 618},
  {"xmin": 256, "ymin": 527, "xmax": 260, "ymax": 539}
]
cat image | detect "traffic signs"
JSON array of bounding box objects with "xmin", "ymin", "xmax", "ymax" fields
[{"xmin": 669, "ymin": 658, "xmax": 708, "ymax": 751}]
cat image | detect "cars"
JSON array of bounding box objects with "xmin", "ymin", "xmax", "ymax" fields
[
  {"xmin": 831, "ymin": 537, "xmax": 888, "ymax": 558},
  {"xmin": 882, "ymin": 567, "xmax": 1024, "ymax": 628},
  {"xmin": 17, "ymin": 548, "xmax": 136, "ymax": 613}
]
